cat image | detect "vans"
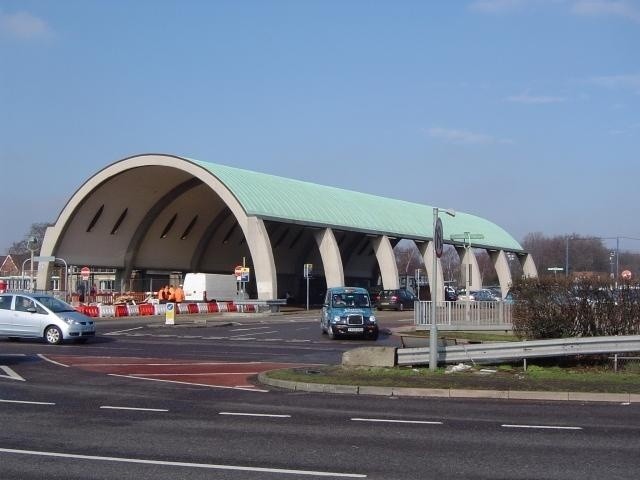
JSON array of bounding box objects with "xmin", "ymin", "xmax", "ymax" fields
[
  {"xmin": 0, "ymin": 293, "xmax": 96, "ymax": 345},
  {"xmin": 320, "ymin": 286, "xmax": 379, "ymax": 342}
]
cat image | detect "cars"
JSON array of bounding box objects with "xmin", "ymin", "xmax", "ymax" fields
[
  {"xmin": 444, "ymin": 283, "xmax": 599, "ymax": 316},
  {"xmin": 375, "ymin": 288, "xmax": 422, "ymax": 312}
]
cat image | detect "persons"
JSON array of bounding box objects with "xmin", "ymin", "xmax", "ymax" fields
[
  {"xmin": 168, "ymin": 285, "xmax": 175, "ymax": 302},
  {"xmin": 335, "ymin": 295, "xmax": 348, "ymax": 306},
  {"xmin": 164, "ymin": 284, "xmax": 171, "ymax": 301},
  {"xmin": 158, "ymin": 286, "xmax": 168, "ymax": 305},
  {"xmin": 174, "ymin": 285, "xmax": 185, "ymax": 302}
]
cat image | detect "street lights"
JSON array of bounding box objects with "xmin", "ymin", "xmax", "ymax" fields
[
  {"xmin": 427, "ymin": 207, "xmax": 455, "ymax": 375},
  {"xmin": 450, "ymin": 229, "xmax": 485, "ymax": 323},
  {"xmin": 548, "ymin": 266, "xmax": 563, "ymax": 285},
  {"xmin": 26, "ymin": 237, "xmax": 40, "ymax": 293}
]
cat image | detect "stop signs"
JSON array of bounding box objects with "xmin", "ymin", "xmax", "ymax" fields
[
  {"xmin": 81, "ymin": 267, "xmax": 91, "ymax": 277},
  {"xmin": 235, "ymin": 266, "xmax": 243, "ymax": 276},
  {"xmin": 621, "ymin": 270, "xmax": 631, "ymax": 281}
]
